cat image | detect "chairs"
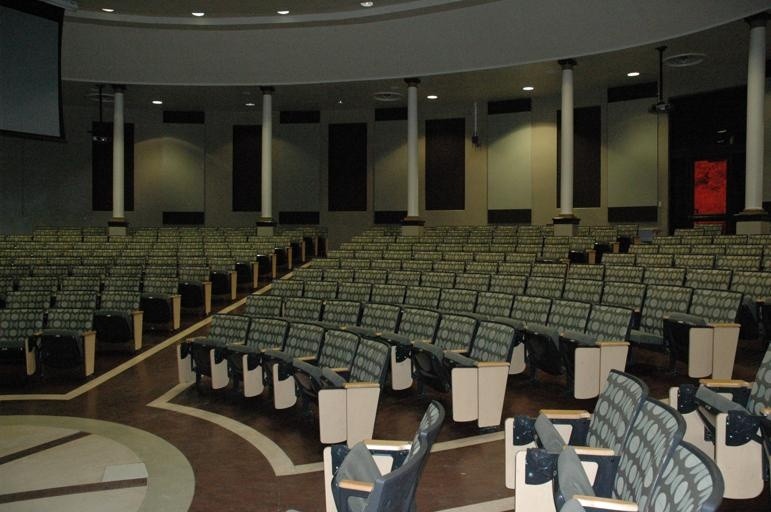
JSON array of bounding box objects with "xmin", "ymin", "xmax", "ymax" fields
[
  {"xmin": 294, "ymin": 329, "xmax": 360, "ymax": 398},
  {"xmin": 305, "ymin": 221, "xmax": 769, "ymax": 510},
  {"xmin": 225, "ymin": 315, "xmax": 288, "ymax": 398},
  {"xmin": 264, "ymin": 321, "xmax": 324, "ymax": 408},
  {"xmin": 32, "ymin": 308, "xmax": 96, "ymax": 376},
  {"xmin": 176, "ymin": 312, "xmax": 250, "ymax": 389},
  {"xmin": 0, "ymin": 309, "xmax": 43, "ymax": 375},
  {"xmin": 0, "ymin": 225, "xmax": 330, "ymax": 350}
]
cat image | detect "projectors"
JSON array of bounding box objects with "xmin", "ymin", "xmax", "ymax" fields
[
  {"xmin": 648, "ymin": 103, "xmax": 675, "ymax": 112},
  {"xmin": 92, "ymin": 135, "xmax": 112, "ymax": 145}
]
